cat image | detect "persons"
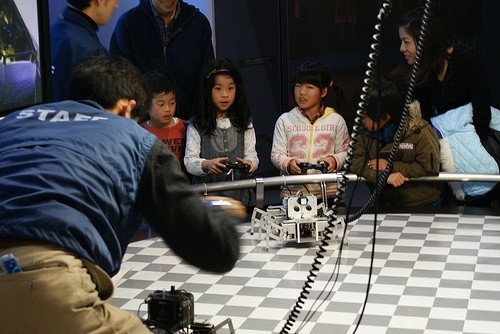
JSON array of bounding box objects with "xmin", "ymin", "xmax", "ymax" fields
[
  {"xmin": 271, "ymin": 62, "xmax": 351, "ymax": 209},
  {"xmin": 388, "ymin": 6, "xmax": 492, "ymax": 216},
  {"xmin": 184, "ymin": 65, "xmax": 259, "ymax": 223},
  {"xmin": 138, "ymin": 71, "xmax": 194, "ymax": 240},
  {"xmin": 350, "ymin": 78, "xmax": 444, "ymax": 214},
  {"xmin": 109, "ymin": 0, "xmax": 216, "ymax": 122},
  {"xmin": 49, "ymin": 0, "xmax": 118, "ymax": 101},
  {"xmin": 0, "ymin": 52, "xmax": 246, "ymax": 334}
]
